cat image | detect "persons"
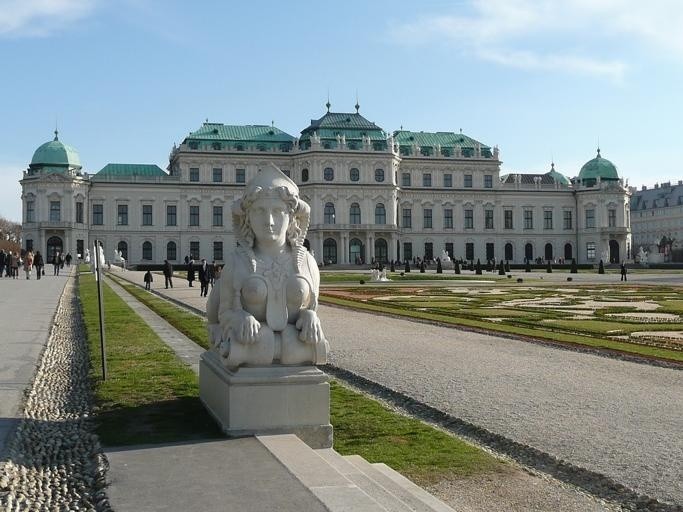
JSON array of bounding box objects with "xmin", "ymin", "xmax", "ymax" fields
[
  {"xmin": 372, "ymin": 255, "xmax": 567, "ymax": 270},
  {"xmin": 143, "ymin": 255, "xmax": 215, "ymax": 297},
  {"xmin": 620, "ymin": 261, "xmax": 627, "ymax": 281},
  {"xmin": 210, "ymin": 162, "xmax": 324, "ymax": 344},
  {"xmin": 1, "ymin": 249, "xmax": 72, "ymax": 278}
]
